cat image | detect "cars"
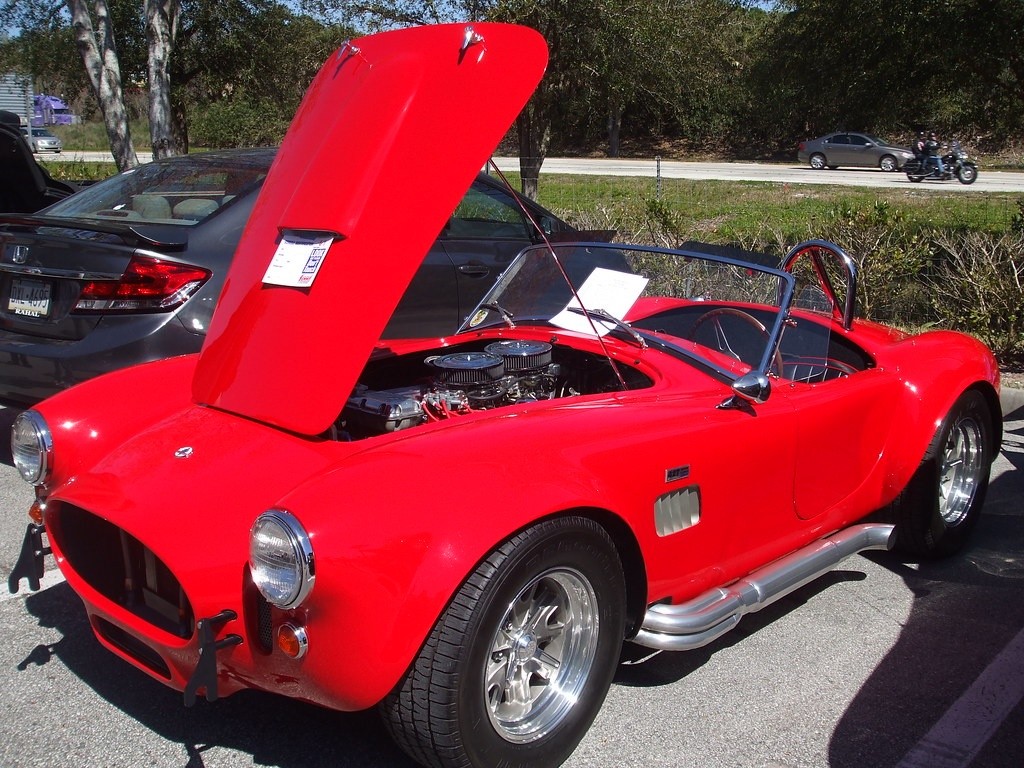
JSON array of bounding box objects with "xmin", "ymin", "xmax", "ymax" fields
[
  {"xmin": 798, "ymin": 131, "xmax": 915, "ymax": 172},
  {"xmin": 19, "ymin": 127, "xmax": 62, "ymax": 153},
  {"xmin": 0, "ymin": 110, "xmax": 631, "ymax": 411}
]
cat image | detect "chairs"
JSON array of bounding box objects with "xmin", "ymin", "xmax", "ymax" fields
[
  {"xmin": 172, "ymin": 199, "xmax": 219, "ymax": 221},
  {"xmin": 132, "ymin": 195, "xmax": 173, "ymax": 220}
]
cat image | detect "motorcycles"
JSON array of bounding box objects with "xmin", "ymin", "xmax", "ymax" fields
[{"xmin": 904, "ymin": 137, "xmax": 978, "ymax": 186}]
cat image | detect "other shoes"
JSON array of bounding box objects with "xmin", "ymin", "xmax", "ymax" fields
[{"xmin": 942, "ymin": 171, "xmax": 948, "ymax": 175}]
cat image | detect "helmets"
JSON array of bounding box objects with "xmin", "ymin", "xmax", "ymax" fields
[
  {"xmin": 918, "ymin": 131, "xmax": 924, "ymax": 136},
  {"xmin": 928, "ymin": 132, "xmax": 935, "ymax": 137}
]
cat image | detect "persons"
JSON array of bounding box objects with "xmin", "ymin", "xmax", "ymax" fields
[
  {"xmin": 925, "ymin": 131, "xmax": 948, "ymax": 173},
  {"xmin": 915, "ymin": 131, "xmax": 930, "ymax": 171}
]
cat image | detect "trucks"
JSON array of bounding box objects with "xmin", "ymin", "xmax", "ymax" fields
[{"xmin": 0, "ymin": 73, "xmax": 74, "ymax": 138}]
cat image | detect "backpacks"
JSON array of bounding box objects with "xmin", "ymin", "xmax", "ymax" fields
[{"xmin": 910, "ymin": 141, "xmax": 923, "ymax": 159}]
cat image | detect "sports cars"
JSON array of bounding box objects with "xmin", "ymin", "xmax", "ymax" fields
[{"xmin": 9, "ymin": 25, "xmax": 1007, "ymax": 768}]
